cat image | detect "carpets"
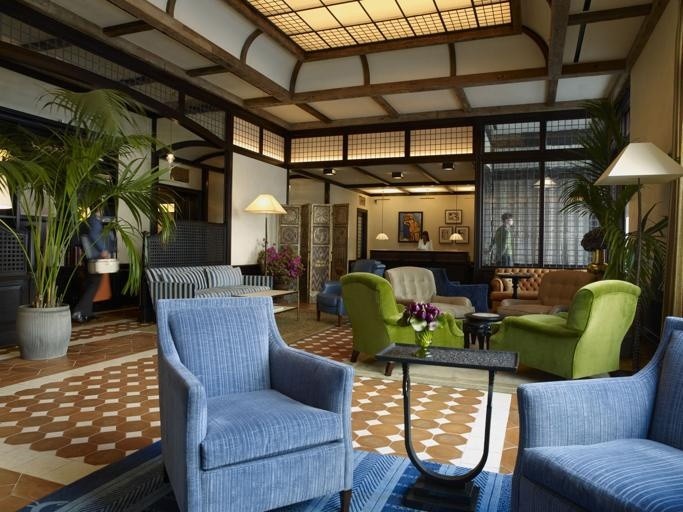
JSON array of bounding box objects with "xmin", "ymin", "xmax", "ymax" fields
[{"xmin": 14, "ymin": 437, "xmax": 514, "ymax": 512}]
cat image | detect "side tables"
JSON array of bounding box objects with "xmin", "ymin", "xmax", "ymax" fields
[{"xmin": 375, "ymin": 341, "xmax": 520, "ymax": 511}]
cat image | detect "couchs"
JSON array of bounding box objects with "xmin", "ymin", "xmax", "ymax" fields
[
  {"xmin": 144, "ymin": 265, "xmax": 271, "ymax": 324},
  {"xmin": 489, "ymin": 266, "xmax": 642, "ymax": 380},
  {"xmin": 514, "ymin": 316, "xmax": 682, "ymax": 512},
  {"xmin": 316, "ymin": 259, "xmax": 500, "ymax": 376},
  {"xmin": 155, "ymin": 296, "xmax": 355, "ymax": 512}
]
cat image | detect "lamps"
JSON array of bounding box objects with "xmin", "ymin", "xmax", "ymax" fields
[
  {"xmin": 590, "ymin": 140, "xmax": 682, "ymax": 377},
  {"xmin": 375, "ymin": 190, "xmax": 389, "ymax": 241},
  {"xmin": 322, "ymin": 168, "xmax": 336, "ymax": 175},
  {"xmin": 391, "ymin": 171, "xmax": 405, "ymax": 180},
  {"xmin": 441, "ymin": 162, "xmax": 456, "ymax": 171},
  {"xmin": 166, "ymin": 119, "xmax": 175, "ymax": 164},
  {"xmin": 242, "ymin": 192, "xmax": 288, "ymax": 275},
  {"xmin": 448, "ymin": 184, "xmax": 464, "ymax": 241}
]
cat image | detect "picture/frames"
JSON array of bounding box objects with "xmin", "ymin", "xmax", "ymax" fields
[{"xmin": 397, "ymin": 209, "xmax": 469, "ymax": 245}]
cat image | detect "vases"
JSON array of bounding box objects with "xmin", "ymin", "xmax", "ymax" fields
[{"xmin": 586, "ymin": 248, "xmax": 611, "ymax": 283}]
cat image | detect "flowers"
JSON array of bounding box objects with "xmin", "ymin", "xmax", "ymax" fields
[
  {"xmin": 580, "ymin": 226, "xmax": 610, "ymax": 252},
  {"xmin": 254, "ymin": 235, "xmax": 307, "ymax": 285}
]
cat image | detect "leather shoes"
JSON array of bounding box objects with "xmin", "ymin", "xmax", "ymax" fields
[{"xmin": 72, "ymin": 312, "xmax": 94, "ymax": 324}]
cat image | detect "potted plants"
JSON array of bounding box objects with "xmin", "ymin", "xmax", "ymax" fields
[
  {"xmin": 607, "ymin": 200, "xmax": 670, "ymax": 360},
  {"xmin": 0, "ymin": 78, "xmax": 193, "ymax": 361}
]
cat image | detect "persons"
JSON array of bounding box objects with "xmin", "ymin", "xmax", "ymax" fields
[
  {"xmin": 488, "ymin": 213, "xmax": 513, "ymax": 266},
  {"xmin": 71, "ymin": 200, "xmax": 109, "ymax": 323},
  {"xmin": 417, "ymin": 230, "xmax": 433, "ymax": 250}
]
